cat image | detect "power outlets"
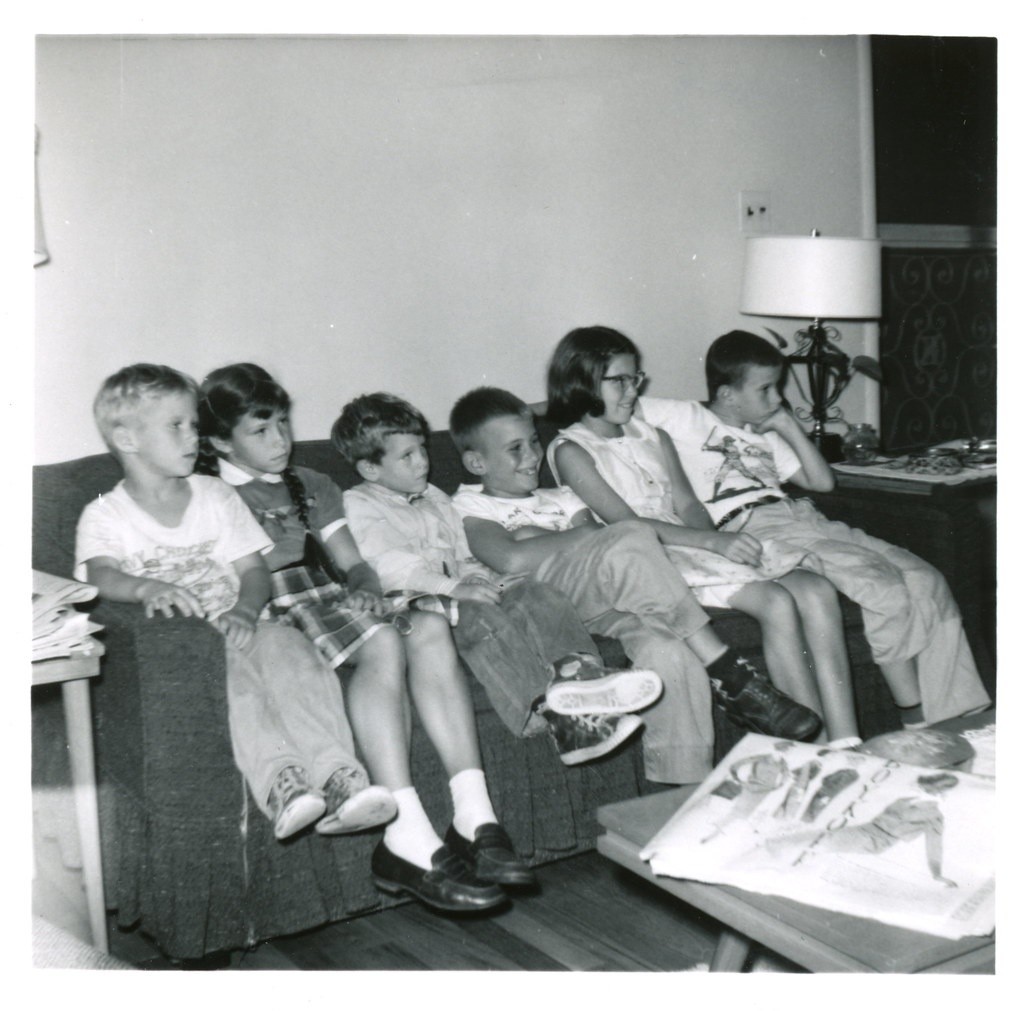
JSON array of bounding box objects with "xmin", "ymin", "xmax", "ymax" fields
[{"xmin": 738, "ymin": 187, "xmax": 772, "ymax": 233}]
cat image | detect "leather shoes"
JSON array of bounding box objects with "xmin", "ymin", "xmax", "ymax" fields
[
  {"xmin": 443, "ymin": 822, "xmax": 536, "ymax": 884},
  {"xmin": 370, "ymin": 838, "xmax": 505, "ymax": 910}
]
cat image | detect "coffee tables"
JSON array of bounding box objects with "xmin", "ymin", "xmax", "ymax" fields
[{"xmin": 595, "ymin": 708, "xmax": 995, "ymax": 973}]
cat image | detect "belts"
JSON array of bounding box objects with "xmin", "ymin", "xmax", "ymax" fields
[{"xmin": 715, "ymin": 495, "xmax": 783, "ymax": 530}]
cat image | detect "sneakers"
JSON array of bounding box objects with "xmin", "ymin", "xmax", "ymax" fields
[
  {"xmin": 546, "ymin": 652, "xmax": 663, "ymax": 715},
  {"xmin": 268, "ymin": 765, "xmax": 327, "ymax": 839},
  {"xmin": 315, "ymin": 766, "xmax": 397, "ymax": 834},
  {"xmin": 709, "ymin": 656, "xmax": 822, "ymax": 743},
  {"xmin": 542, "ymin": 707, "xmax": 643, "ymax": 765}
]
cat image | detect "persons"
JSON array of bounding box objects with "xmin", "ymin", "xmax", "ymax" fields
[{"xmin": 71, "ymin": 325, "xmax": 992, "ymax": 914}]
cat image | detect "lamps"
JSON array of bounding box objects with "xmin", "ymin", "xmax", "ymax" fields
[{"xmin": 738, "ymin": 227, "xmax": 883, "ymax": 465}]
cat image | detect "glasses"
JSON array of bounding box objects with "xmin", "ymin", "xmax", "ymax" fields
[{"xmin": 601, "ymin": 371, "xmax": 645, "ymax": 389}]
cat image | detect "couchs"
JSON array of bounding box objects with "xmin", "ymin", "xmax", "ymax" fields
[{"xmin": 31, "ymin": 413, "xmax": 902, "ymax": 970}]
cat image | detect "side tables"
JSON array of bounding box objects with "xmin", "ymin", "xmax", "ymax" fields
[
  {"xmin": 781, "ymin": 440, "xmax": 995, "ymax": 712},
  {"xmin": 31, "ymin": 652, "xmax": 108, "ymax": 956}
]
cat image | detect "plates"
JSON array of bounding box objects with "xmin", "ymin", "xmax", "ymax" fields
[{"xmin": 894, "ymin": 447, "xmax": 959, "ymax": 457}]
